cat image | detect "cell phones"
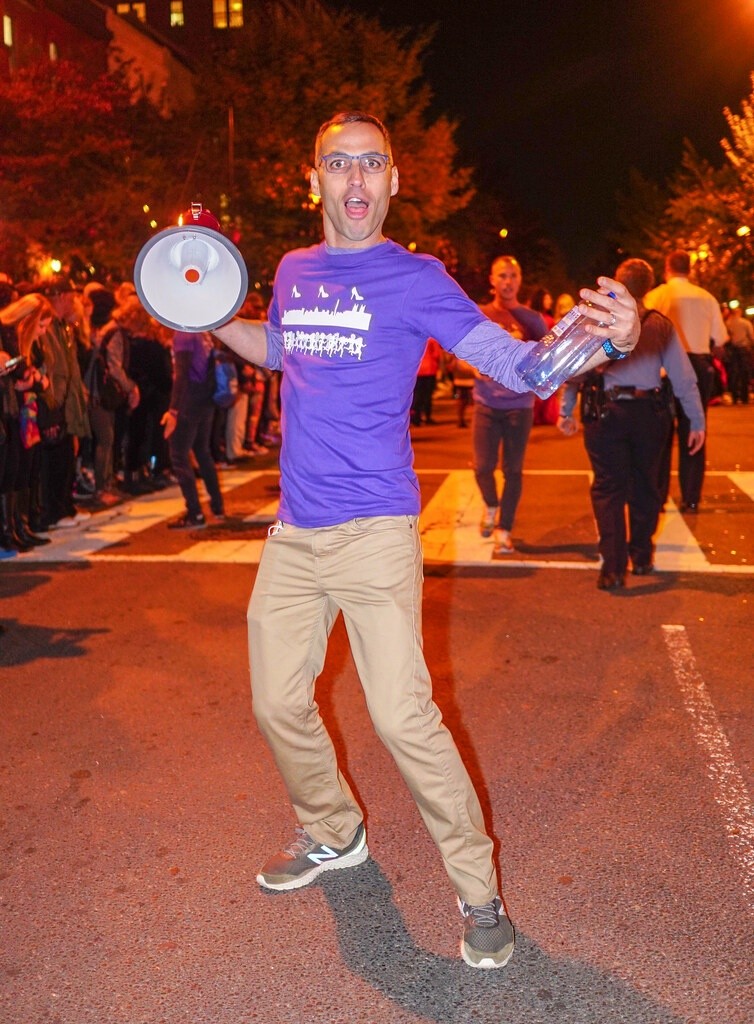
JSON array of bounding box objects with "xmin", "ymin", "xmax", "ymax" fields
[{"xmin": 5, "ymin": 355, "xmax": 24, "ymax": 368}]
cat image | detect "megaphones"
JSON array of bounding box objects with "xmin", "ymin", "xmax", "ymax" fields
[{"xmin": 132, "ymin": 201, "xmax": 248, "ymax": 334}]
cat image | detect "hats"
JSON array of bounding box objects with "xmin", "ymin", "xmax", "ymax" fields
[{"xmin": 46, "ymin": 275, "xmax": 74, "ymax": 297}]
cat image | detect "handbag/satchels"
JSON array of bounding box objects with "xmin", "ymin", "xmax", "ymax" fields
[
  {"xmin": 96, "ymin": 330, "xmax": 126, "ymax": 410},
  {"xmin": 40, "ymin": 408, "xmax": 67, "ymax": 444}
]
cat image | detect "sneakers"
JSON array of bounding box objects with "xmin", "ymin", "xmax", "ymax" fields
[
  {"xmin": 257, "ymin": 824, "xmax": 376, "ymax": 891},
  {"xmin": 457, "ymin": 891, "xmax": 514, "ymax": 972}
]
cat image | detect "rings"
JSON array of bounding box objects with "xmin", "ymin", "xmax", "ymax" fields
[{"xmin": 609, "ymin": 314, "xmax": 616, "ymax": 324}]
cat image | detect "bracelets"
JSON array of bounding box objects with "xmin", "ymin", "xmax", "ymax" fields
[{"xmin": 603, "ymin": 339, "xmax": 631, "ymax": 360}]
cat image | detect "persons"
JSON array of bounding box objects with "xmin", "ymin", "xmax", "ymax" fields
[
  {"xmin": 557, "ymin": 258, "xmax": 705, "ymax": 587},
  {"xmin": 643, "ymin": 250, "xmax": 730, "ymax": 515},
  {"xmin": 207, "ymin": 114, "xmax": 640, "ymax": 968},
  {"xmin": 464, "ymin": 255, "xmax": 546, "ymax": 555},
  {"xmin": 0, "ymin": 274, "xmax": 282, "ymax": 560},
  {"xmin": 409, "ymin": 290, "xmax": 754, "ymax": 428}
]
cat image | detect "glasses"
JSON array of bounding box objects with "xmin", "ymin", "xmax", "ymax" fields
[{"xmin": 314, "ymin": 153, "xmax": 391, "ymax": 173}]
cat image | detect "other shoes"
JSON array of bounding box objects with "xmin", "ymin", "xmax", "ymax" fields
[
  {"xmin": 217, "ymin": 440, "xmax": 269, "ymax": 467},
  {"xmin": 597, "ymin": 571, "xmax": 622, "ymax": 592},
  {"xmin": 57, "ymin": 517, "xmax": 78, "ymax": 526},
  {"xmin": 633, "ymin": 556, "xmax": 653, "ymax": 576},
  {"xmin": 73, "ymin": 512, "xmax": 91, "ymax": 522},
  {"xmin": 168, "ymin": 512, "xmax": 207, "ymax": 530},
  {"xmin": 211, "ymin": 508, "xmax": 224, "ymax": 518},
  {"xmin": 482, "ymin": 505, "xmax": 500, "ymax": 539},
  {"xmin": 680, "ymin": 498, "xmax": 698, "ymax": 513},
  {"xmin": 74, "ymin": 474, "xmax": 173, "ymax": 507},
  {"xmin": 494, "ymin": 526, "xmax": 514, "ymax": 554}
]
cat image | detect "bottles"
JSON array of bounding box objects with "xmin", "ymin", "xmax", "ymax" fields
[{"xmin": 515, "ymin": 291, "xmax": 617, "ymax": 400}]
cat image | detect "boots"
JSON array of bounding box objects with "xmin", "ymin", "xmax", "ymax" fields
[
  {"xmin": 0, "ymin": 491, "xmax": 33, "ymax": 554},
  {"xmin": 15, "ymin": 489, "xmax": 51, "ymax": 546}
]
